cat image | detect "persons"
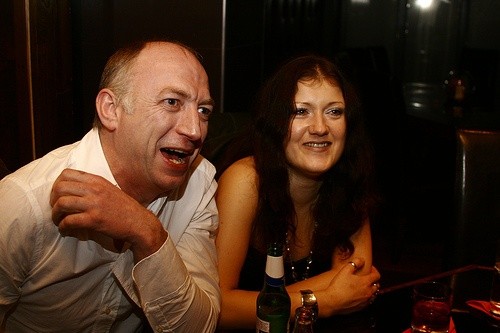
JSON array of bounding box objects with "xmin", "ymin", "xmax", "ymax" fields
[
  {"xmin": 0, "ymin": 38, "xmax": 220, "ymax": 333},
  {"xmin": 216, "ymin": 56, "xmax": 380, "ymax": 329}
]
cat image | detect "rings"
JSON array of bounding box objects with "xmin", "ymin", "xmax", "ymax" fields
[
  {"xmin": 374, "ymin": 284, "xmax": 379, "ymax": 292},
  {"xmin": 348, "ymin": 262, "xmax": 357, "ymax": 268}
]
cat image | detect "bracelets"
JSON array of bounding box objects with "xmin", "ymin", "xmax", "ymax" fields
[{"xmin": 299, "ymin": 289, "xmax": 318, "ymax": 319}]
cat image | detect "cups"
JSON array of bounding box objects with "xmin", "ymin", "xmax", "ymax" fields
[
  {"xmin": 409, "ymin": 278, "xmax": 455, "ymax": 333},
  {"xmin": 488, "ymin": 263, "xmax": 500, "ymax": 316}
]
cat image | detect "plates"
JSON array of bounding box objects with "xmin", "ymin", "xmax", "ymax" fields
[
  {"xmin": 464, "ymin": 299, "xmax": 500, "ymax": 322},
  {"xmin": 399, "ymin": 314, "xmax": 457, "ymax": 333}
]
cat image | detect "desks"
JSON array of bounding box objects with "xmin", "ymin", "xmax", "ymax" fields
[{"xmin": 211, "ymin": 264, "xmax": 500, "ymax": 333}]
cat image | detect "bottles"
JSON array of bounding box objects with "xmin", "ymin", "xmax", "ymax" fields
[
  {"xmin": 290, "ymin": 307, "xmax": 315, "ymax": 333},
  {"xmin": 254, "ymin": 241, "xmax": 291, "ymax": 333},
  {"xmin": 437, "ymin": 63, "xmax": 475, "ymax": 120}
]
